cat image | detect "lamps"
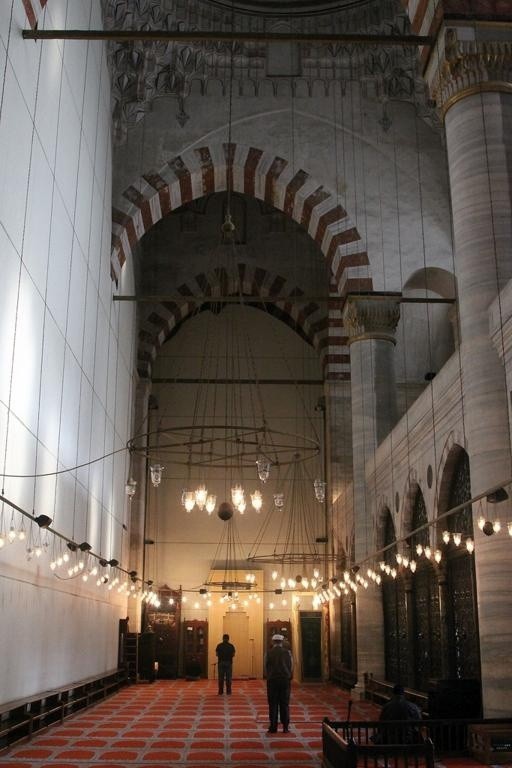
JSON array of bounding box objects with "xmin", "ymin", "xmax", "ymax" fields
[
  {"xmin": 127, "ymin": 37, "xmax": 320, "ymax": 514},
  {"xmin": 246, "ymin": 38, "xmax": 348, "ymax": 590},
  {"xmin": 197, "ymin": 38, "xmax": 261, "ymax": 599},
  {"xmin": 114, "ymin": 561, "xmax": 158, "ymax": 599}
]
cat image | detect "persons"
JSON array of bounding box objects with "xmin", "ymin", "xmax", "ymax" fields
[
  {"xmin": 263, "ymin": 635, "xmax": 294, "ymax": 734},
  {"xmin": 216, "ymin": 633, "xmax": 235, "ymax": 695},
  {"xmin": 279, "ymin": 637, "xmax": 294, "ymax": 690},
  {"xmin": 378, "ymin": 683, "xmax": 422, "ymax": 748}
]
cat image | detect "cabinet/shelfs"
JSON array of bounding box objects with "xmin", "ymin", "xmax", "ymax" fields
[{"xmin": 183, "ymin": 621, "xmax": 208, "ymax": 676}]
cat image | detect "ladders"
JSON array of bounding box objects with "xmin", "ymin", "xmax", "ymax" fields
[{"xmin": 123, "ymin": 630, "xmax": 139, "ymax": 687}]
